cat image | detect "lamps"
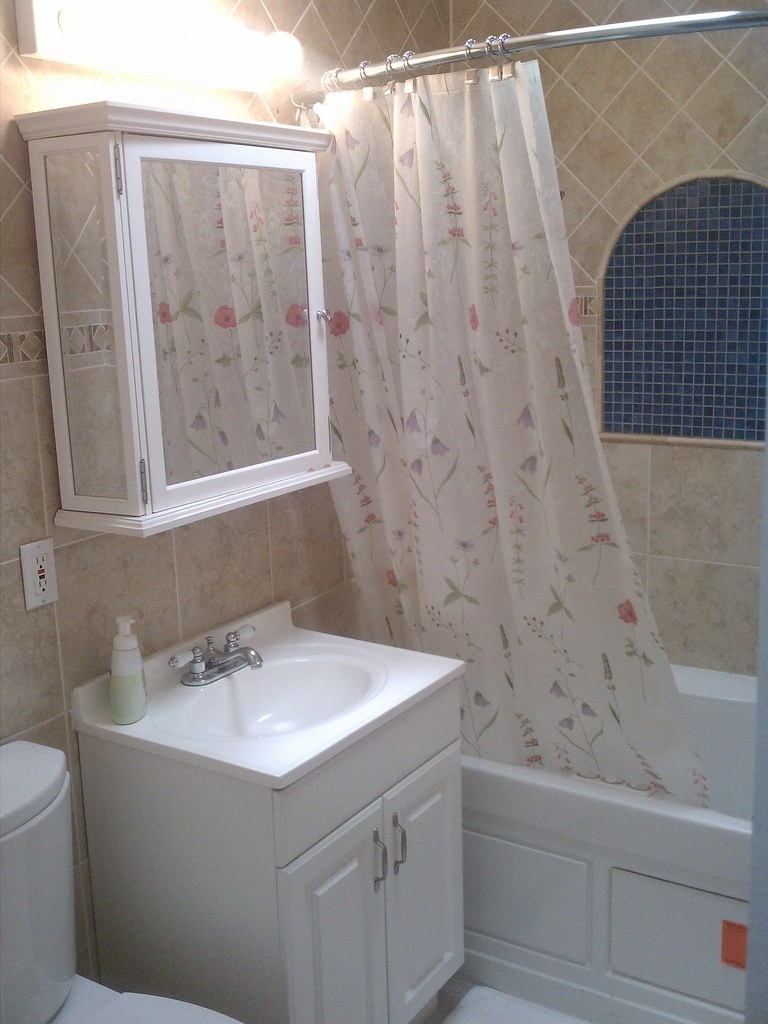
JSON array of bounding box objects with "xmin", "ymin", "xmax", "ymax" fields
[{"xmin": 15, "ymin": 0, "xmax": 305, "ymax": 98}]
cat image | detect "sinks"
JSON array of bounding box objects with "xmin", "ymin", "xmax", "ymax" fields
[{"xmin": 174, "ymin": 643, "xmax": 389, "ymax": 738}]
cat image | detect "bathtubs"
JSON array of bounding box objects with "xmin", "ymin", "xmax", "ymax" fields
[{"xmin": 461, "ymin": 662, "xmax": 758, "ymax": 1024}]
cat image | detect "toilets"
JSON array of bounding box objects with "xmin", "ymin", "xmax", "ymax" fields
[{"xmin": 0, "ymin": 739, "xmax": 251, "ymax": 1024}]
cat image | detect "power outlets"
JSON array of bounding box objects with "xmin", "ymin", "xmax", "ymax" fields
[{"xmin": 19, "ymin": 538, "xmax": 60, "ymax": 612}]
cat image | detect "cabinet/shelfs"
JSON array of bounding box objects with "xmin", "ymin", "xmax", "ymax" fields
[
  {"xmin": 76, "ymin": 677, "xmax": 467, "ymax": 1023},
  {"xmin": 15, "ymin": 98, "xmax": 358, "ymax": 536}
]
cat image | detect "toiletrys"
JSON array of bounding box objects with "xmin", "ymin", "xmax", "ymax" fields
[{"xmin": 108, "ymin": 616, "xmax": 149, "ymax": 725}]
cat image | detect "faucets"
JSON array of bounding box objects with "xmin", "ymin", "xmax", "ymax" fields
[{"xmin": 200, "ymin": 634, "xmax": 265, "ymax": 671}]
